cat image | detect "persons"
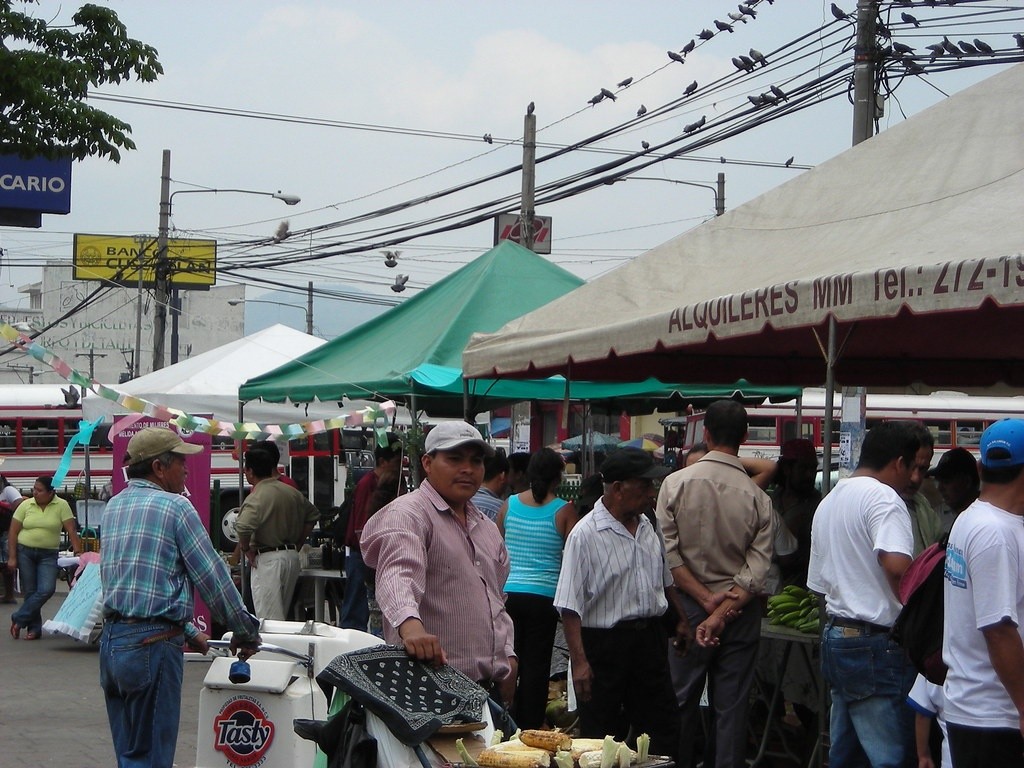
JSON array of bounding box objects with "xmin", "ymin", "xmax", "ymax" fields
[
  {"xmin": 942, "ymin": 418, "xmax": 1024, "ymax": 768},
  {"xmin": 773, "ymin": 440, "xmax": 823, "ymax": 591},
  {"xmin": 361, "ymin": 420, "xmax": 516, "ymax": 718},
  {"xmin": 896, "ymin": 421, "xmax": 950, "ymax": 561},
  {"xmin": 471, "ymin": 451, "xmax": 508, "ymax": 521},
  {"xmin": 806, "ymin": 422, "xmax": 914, "ymax": 767},
  {"xmin": 686, "ymin": 443, "xmax": 779, "ymax": 491},
  {"xmin": 234, "ymin": 441, "xmax": 322, "ymax": 622},
  {"xmin": 339, "ymin": 432, "xmax": 407, "ymax": 633},
  {"xmin": 8, "ymin": 476, "xmax": 82, "ymax": 639},
  {"xmin": 554, "ymin": 446, "xmax": 681, "ymax": 767},
  {"xmin": 98, "ymin": 428, "xmax": 261, "ymax": 768},
  {"xmin": 908, "ymin": 646, "xmax": 952, "ymax": 768},
  {"xmin": 498, "ymin": 448, "xmax": 576, "ymax": 730},
  {"xmin": 0, "ymin": 474, "xmax": 23, "ymax": 603},
  {"xmin": 656, "ymin": 400, "xmax": 772, "ymax": 768},
  {"xmin": 929, "ymin": 446, "xmax": 982, "ymax": 518}
]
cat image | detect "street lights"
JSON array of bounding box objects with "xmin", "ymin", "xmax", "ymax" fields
[
  {"xmin": 151, "ymin": 148, "xmax": 303, "ymax": 369},
  {"xmin": 225, "ymin": 281, "xmax": 313, "ymax": 336},
  {"xmin": 607, "ymin": 171, "xmax": 726, "ymax": 215}
]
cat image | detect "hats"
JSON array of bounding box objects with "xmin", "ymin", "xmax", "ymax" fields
[
  {"xmin": 979, "ymin": 416, "xmax": 1024, "ymax": 469},
  {"xmin": 927, "ymin": 447, "xmax": 979, "ymax": 483},
  {"xmin": 127, "ymin": 427, "xmax": 204, "ymax": 465},
  {"xmin": 424, "ymin": 421, "xmax": 496, "ymax": 462},
  {"xmin": 598, "ymin": 446, "xmax": 672, "ymax": 482}
]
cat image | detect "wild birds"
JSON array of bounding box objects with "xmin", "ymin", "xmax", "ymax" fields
[
  {"xmin": 876, "ymin": 41, "xmax": 925, "ymax": 75},
  {"xmin": 747, "ymin": 85, "xmax": 789, "ymax": 108},
  {"xmin": 60, "ymin": 384, "xmax": 81, "ymax": 406},
  {"xmin": 901, "ymin": 12, "xmax": 921, "ymax": 28},
  {"xmin": 894, "ymin": 0, "xmax": 915, "ymax": 8},
  {"xmin": 641, "ymin": 141, "xmax": 649, "ymax": 150},
  {"xmin": 379, "ymin": 250, "xmax": 403, "ymax": 267},
  {"xmin": 587, "ymin": 88, "xmax": 617, "ymax": 107},
  {"xmin": 616, "ymin": 76, "xmax": 633, "ymax": 88},
  {"xmin": 637, "ymin": 104, "xmax": 646, "ymax": 117},
  {"xmin": 273, "ymin": 220, "xmax": 291, "ymax": 244},
  {"xmin": 682, "ymin": 79, "xmax": 698, "ymax": 96},
  {"xmin": 925, "ymin": 34, "xmax": 994, "ymax": 58},
  {"xmin": 1012, "ymin": 33, "xmax": 1024, "ymax": 48},
  {"xmin": 830, "ymin": 2, "xmax": 850, "ymax": 20},
  {"xmin": 483, "ymin": 133, "xmax": 492, "ymax": 144},
  {"xmin": 732, "ymin": 48, "xmax": 768, "ymax": 73},
  {"xmin": 390, "ymin": 273, "xmax": 409, "ymax": 292},
  {"xmin": 924, "ymin": 0, "xmax": 969, "ymax": 6},
  {"xmin": 682, "ymin": 115, "xmax": 706, "ymax": 133},
  {"xmin": 666, "ymin": 0, "xmax": 776, "ymax": 65},
  {"xmin": 784, "ymin": 156, "xmax": 794, "ymax": 167}
]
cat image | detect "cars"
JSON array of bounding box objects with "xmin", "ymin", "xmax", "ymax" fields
[{"xmin": 484, "ymin": 423, "xmax": 512, "ymax": 458}]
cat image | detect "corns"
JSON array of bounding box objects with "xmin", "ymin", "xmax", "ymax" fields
[{"xmin": 471, "ymin": 729, "xmax": 638, "ymax": 768}]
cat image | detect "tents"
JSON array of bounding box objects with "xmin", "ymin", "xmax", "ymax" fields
[
  {"xmin": 81, "ymin": 323, "xmax": 438, "ymax": 551},
  {"xmin": 461, "ymin": 58, "xmax": 1024, "ymax": 498},
  {"xmin": 239, "ymin": 239, "xmax": 802, "ymax": 448}
]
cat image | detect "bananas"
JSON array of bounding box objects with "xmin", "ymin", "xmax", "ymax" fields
[{"xmin": 767, "ymin": 585, "xmax": 822, "ymax": 632}]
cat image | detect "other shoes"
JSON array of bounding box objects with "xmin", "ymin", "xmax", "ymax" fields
[
  {"xmin": 11, "ymin": 615, "xmax": 21, "ymax": 639},
  {"xmin": 3, "ymin": 597, "xmax": 17, "ymax": 605},
  {"xmin": 23, "ymin": 631, "xmax": 40, "ymax": 639}
]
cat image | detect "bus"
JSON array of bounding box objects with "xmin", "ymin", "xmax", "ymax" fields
[
  {"xmin": 679, "ymin": 387, "xmax": 1024, "ymax": 494},
  {"xmin": 0, "ymin": 384, "xmax": 382, "ymax": 554}
]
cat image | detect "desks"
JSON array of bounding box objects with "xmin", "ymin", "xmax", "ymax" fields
[
  {"xmin": 300, "ymin": 570, "xmax": 347, "ymax": 621},
  {"xmin": 750, "ymin": 619, "xmax": 829, "ymax": 768}
]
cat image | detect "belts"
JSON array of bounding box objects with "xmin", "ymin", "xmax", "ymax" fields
[
  {"xmin": 106, "ymin": 615, "xmax": 187, "ymax": 625},
  {"xmin": 256, "ymin": 545, "xmax": 298, "ymax": 555},
  {"xmin": 828, "ymin": 616, "xmax": 892, "ymax": 634}
]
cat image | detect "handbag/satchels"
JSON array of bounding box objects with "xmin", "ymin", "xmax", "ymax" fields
[
  {"xmin": 73, "ymin": 468, "xmax": 87, "ymax": 499},
  {"xmin": 80, "ymin": 527, "xmax": 99, "ymax": 553}
]
